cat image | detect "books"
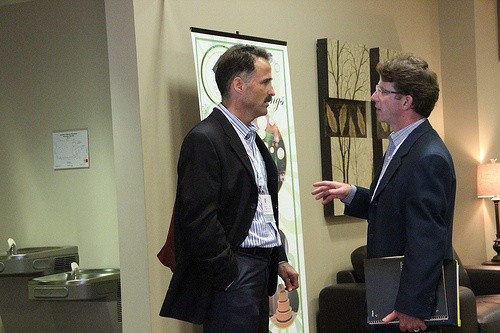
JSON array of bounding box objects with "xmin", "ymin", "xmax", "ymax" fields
[{"xmin": 364, "ymin": 254, "xmax": 461, "ymax": 328}]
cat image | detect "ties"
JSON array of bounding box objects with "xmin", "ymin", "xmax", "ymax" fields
[{"xmin": 383, "ymin": 139, "xmax": 396, "ymax": 168}]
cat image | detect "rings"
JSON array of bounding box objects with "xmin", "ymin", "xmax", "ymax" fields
[{"xmin": 414, "ymin": 328, "xmax": 419, "ymax": 332}]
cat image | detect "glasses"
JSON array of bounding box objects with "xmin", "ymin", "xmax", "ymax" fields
[{"xmin": 375, "ymin": 84, "xmax": 407, "ymax": 96}]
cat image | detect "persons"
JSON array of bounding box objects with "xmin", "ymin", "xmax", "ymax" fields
[
  {"xmin": 159, "ymin": 44, "xmax": 299, "ymax": 333},
  {"xmin": 311, "ymin": 53, "xmax": 457, "ymax": 333}
]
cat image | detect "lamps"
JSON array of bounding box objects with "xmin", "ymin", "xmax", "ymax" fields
[{"xmin": 475, "ymin": 159, "xmax": 500, "ymax": 266}]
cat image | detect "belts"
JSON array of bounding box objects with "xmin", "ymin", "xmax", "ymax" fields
[{"xmin": 239, "ymin": 248, "xmax": 275, "ymax": 256}]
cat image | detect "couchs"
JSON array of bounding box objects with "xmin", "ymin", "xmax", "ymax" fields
[{"xmin": 318, "ymin": 241, "xmax": 500, "ymax": 333}]
